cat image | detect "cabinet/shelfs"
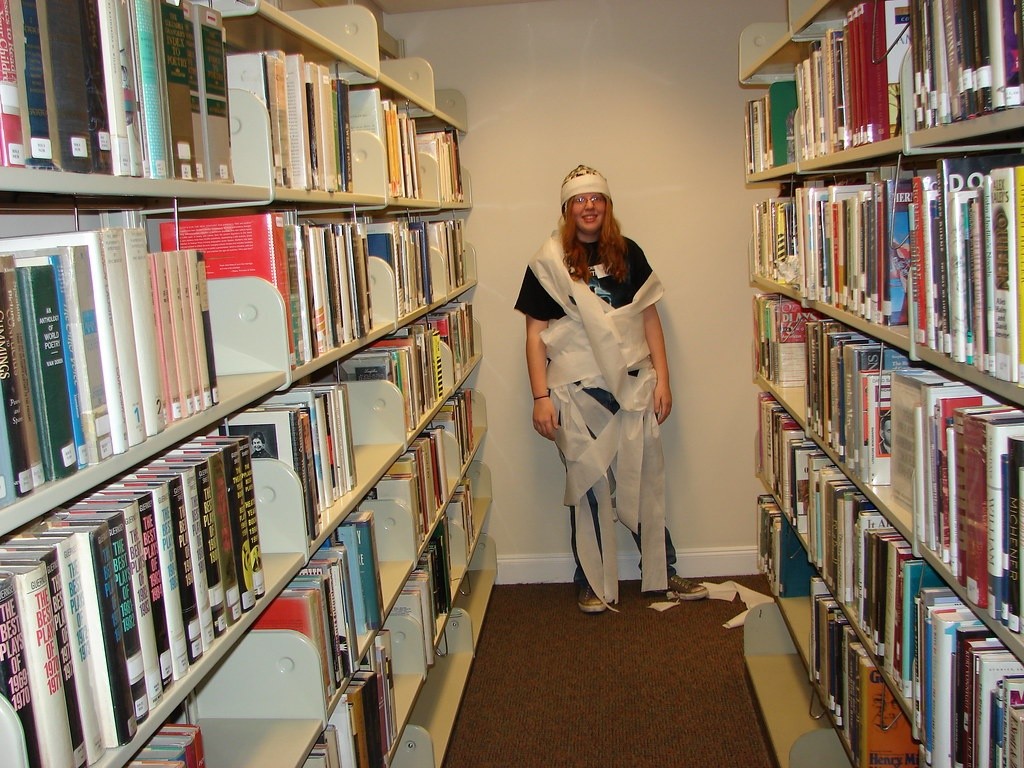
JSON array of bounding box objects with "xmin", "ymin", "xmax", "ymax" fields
[
  {"xmin": 0, "ymin": 0, "xmax": 497, "ymax": 768},
  {"xmin": 742, "ymin": 0, "xmax": 1024, "ymax": 768}
]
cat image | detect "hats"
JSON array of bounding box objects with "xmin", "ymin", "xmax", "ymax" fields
[{"xmin": 559, "ymin": 164, "xmax": 614, "ymax": 219}]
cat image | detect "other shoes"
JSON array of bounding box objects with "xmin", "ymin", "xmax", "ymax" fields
[
  {"xmin": 578, "ymin": 581, "xmax": 607, "ymax": 613},
  {"xmin": 654, "ymin": 574, "xmax": 709, "ymax": 600}
]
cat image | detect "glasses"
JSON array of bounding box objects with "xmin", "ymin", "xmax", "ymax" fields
[{"xmin": 573, "ymin": 196, "xmax": 605, "ymax": 204}]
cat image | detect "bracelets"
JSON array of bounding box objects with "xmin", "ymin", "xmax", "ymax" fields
[{"xmin": 534, "ymin": 395, "xmax": 550, "ymax": 400}]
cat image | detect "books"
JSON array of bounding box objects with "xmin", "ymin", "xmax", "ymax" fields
[
  {"xmin": 0, "ymin": 0, "xmax": 475, "ymax": 768},
  {"xmin": 742, "ymin": 0, "xmax": 1024, "ymax": 768}
]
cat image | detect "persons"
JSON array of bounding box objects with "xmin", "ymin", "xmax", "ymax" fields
[
  {"xmin": 513, "ymin": 165, "xmax": 709, "ymax": 614},
  {"xmin": 877, "ymin": 411, "xmax": 892, "ymax": 455}
]
